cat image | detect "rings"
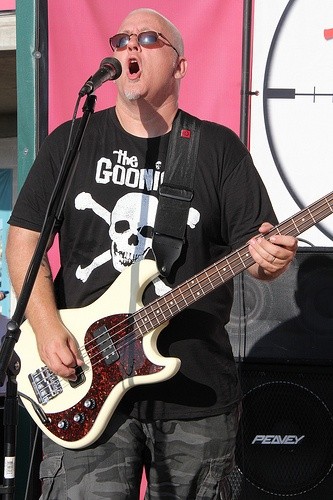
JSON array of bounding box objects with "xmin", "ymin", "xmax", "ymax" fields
[{"xmin": 271, "ymin": 257, "xmax": 276, "ymax": 264}]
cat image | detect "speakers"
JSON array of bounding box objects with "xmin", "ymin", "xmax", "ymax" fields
[
  {"xmin": 224, "ymin": 246, "xmax": 333, "ymax": 365},
  {"xmin": 236, "ymin": 364, "xmax": 333, "ymax": 500}
]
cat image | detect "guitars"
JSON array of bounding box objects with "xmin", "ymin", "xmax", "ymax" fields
[{"xmin": 14, "ymin": 190, "xmax": 333, "ymax": 450}]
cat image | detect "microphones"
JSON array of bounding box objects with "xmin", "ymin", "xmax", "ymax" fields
[{"xmin": 78, "ymin": 57, "xmax": 122, "ymax": 96}]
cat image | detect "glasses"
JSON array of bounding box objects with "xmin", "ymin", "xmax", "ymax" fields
[{"xmin": 110, "ymin": 31, "xmax": 179, "ymax": 56}]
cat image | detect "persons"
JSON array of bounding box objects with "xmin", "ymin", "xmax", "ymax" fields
[{"xmin": 5, "ymin": 9, "xmax": 300, "ymax": 500}]
